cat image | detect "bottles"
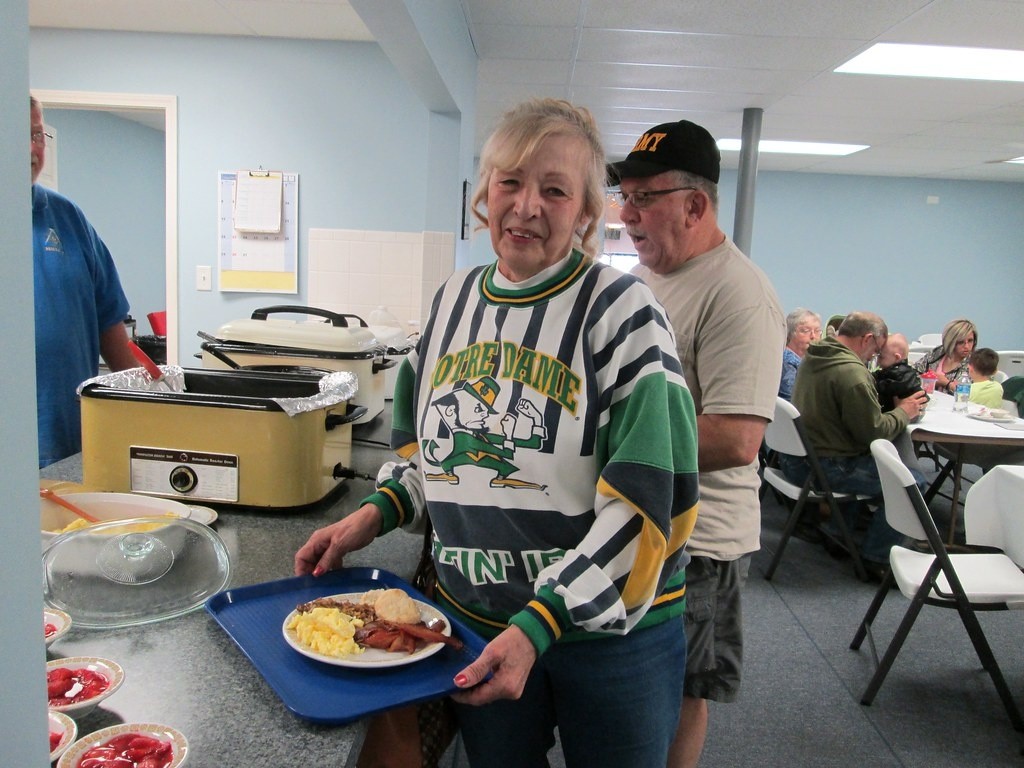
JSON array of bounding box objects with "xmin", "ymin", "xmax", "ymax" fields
[
  {"xmin": 952, "ymin": 371, "xmax": 973, "ymax": 415},
  {"xmin": 920, "ymin": 370, "xmax": 938, "ymax": 394}
]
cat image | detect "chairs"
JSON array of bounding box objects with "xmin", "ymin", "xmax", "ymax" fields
[
  {"xmin": 996, "ymin": 351, "xmax": 1024, "ymax": 377},
  {"xmin": 759, "ymin": 397, "xmax": 872, "ymax": 584},
  {"xmin": 849, "ymin": 439, "xmax": 1024, "ymax": 732}
]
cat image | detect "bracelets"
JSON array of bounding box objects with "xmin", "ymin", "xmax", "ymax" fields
[{"xmin": 945, "ymin": 380, "xmax": 953, "ymax": 390}]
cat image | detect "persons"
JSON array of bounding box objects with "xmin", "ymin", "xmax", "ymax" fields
[
  {"xmin": 30, "ymin": 95, "xmax": 141, "ymax": 469},
  {"xmin": 913, "ymin": 318, "xmax": 1004, "ymax": 410},
  {"xmin": 606, "ymin": 120, "xmax": 787, "ymax": 768},
  {"xmin": 294, "ymin": 97, "xmax": 701, "ymax": 768},
  {"xmin": 777, "ymin": 308, "xmax": 930, "ymax": 585}
]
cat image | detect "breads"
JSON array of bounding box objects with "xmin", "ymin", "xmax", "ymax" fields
[{"xmin": 361, "ymin": 588, "xmax": 422, "ymax": 623}]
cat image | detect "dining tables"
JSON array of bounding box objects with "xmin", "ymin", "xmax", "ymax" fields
[{"xmin": 895, "ymin": 389, "xmax": 1024, "ymax": 569}]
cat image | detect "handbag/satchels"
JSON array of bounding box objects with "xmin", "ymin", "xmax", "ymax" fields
[{"xmin": 412, "ymin": 554, "xmax": 437, "ymax": 600}]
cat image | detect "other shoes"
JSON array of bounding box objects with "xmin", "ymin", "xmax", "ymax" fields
[{"xmin": 856, "ymin": 558, "xmax": 899, "ymax": 588}]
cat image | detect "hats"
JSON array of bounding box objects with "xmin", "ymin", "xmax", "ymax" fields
[{"xmin": 604, "ymin": 120, "xmax": 721, "ymax": 188}]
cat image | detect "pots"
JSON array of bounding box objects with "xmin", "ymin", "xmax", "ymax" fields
[
  {"xmin": 192, "ymin": 304, "xmax": 412, "ymax": 433},
  {"xmin": 78, "ymin": 365, "xmax": 371, "ymax": 518}
]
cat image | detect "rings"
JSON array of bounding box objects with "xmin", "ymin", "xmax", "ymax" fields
[
  {"xmin": 920, "ymin": 405, "xmax": 923, "ymax": 410},
  {"xmin": 919, "ymin": 411, "xmax": 922, "ymax": 416}
]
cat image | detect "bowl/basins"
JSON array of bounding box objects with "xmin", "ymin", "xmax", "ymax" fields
[
  {"xmin": 41, "ymin": 491, "xmax": 192, "ymax": 580},
  {"xmin": 47, "ymin": 709, "xmax": 78, "ymax": 762},
  {"xmin": 46, "ymin": 655, "xmax": 126, "ymax": 720},
  {"xmin": 43, "ymin": 607, "xmax": 73, "ymax": 649},
  {"xmin": 55, "ymin": 721, "xmax": 190, "ymax": 768},
  {"xmin": 990, "ymin": 411, "xmax": 1008, "ymax": 417}
]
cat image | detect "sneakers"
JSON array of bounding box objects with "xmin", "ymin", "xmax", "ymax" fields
[{"xmin": 784, "ymin": 515, "xmax": 827, "ymax": 542}]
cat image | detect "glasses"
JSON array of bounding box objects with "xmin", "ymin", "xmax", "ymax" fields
[
  {"xmin": 796, "ymin": 328, "xmax": 823, "ymax": 335},
  {"xmin": 861, "ymin": 335, "xmax": 881, "ymax": 357},
  {"xmin": 31, "ymin": 133, "xmax": 53, "ymax": 146},
  {"xmin": 614, "ymin": 186, "xmax": 696, "ymax": 208},
  {"xmin": 957, "ymin": 339, "xmax": 976, "ymax": 345}
]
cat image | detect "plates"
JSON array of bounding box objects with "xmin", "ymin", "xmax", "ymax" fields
[
  {"xmin": 184, "ymin": 504, "xmax": 219, "ymax": 525},
  {"xmin": 966, "ymin": 413, "xmax": 1016, "ymax": 423},
  {"xmin": 282, "ymin": 591, "xmax": 453, "ymax": 669}
]
